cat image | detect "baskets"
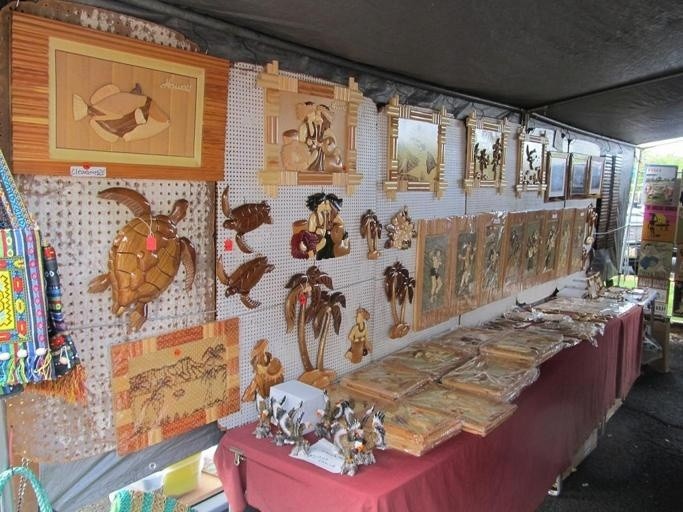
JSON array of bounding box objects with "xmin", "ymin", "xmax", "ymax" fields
[{"xmin": 1, "ymin": 465, "xmax": 197, "ymax": 512}]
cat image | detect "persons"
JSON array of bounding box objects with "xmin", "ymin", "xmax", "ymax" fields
[
  {"xmin": 297, "ymin": 102, "xmax": 341, "ymax": 174},
  {"xmin": 430, "ymin": 222, "xmax": 581, "ymax": 293},
  {"xmin": 348, "ymin": 306, "xmax": 372, "ymax": 363}
]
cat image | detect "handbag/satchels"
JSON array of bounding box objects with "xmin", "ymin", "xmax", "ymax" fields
[{"xmin": 0, "ymin": 228, "xmax": 87, "ymax": 403}]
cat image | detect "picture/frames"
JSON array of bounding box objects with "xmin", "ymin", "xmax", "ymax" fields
[
  {"xmin": 461, "ymin": 108, "xmax": 513, "ymax": 198},
  {"xmin": 9, "ymin": 12, "xmax": 229, "ymax": 181},
  {"xmin": 255, "ymin": 59, "xmax": 364, "ymax": 197},
  {"xmin": 403, "ymin": 206, "xmax": 602, "ymax": 332},
  {"xmin": 588, "ymin": 154, "xmax": 602, "ymax": 199},
  {"xmin": 513, "ymin": 125, "xmax": 548, "ymax": 200},
  {"xmin": 383, "ymin": 95, "xmax": 455, "ymax": 202},
  {"xmin": 569, "ymin": 152, "xmax": 591, "ymax": 199},
  {"xmin": 544, "ymin": 150, "xmax": 570, "ymax": 204}
]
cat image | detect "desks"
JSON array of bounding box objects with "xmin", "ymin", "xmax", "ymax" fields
[{"xmin": 218, "ymin": 284, "xmax": 643, "ymax": 510}]
